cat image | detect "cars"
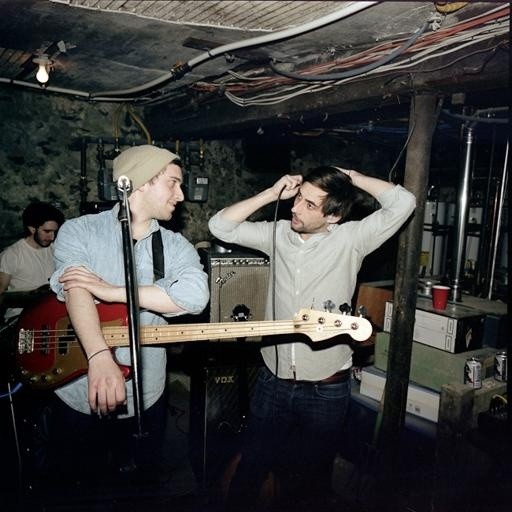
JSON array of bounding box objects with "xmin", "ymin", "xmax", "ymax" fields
[{"xmin": 431, "ymin": 285, "xmax": 450, "ymax": 311}]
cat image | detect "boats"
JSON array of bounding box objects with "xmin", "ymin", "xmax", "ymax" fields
[
  {"xmin": 187, "ymin": 356, "xmax": 251, "ymax": 490},
  {"xmin": 201, "ymin": 249, "xmax": 271, "ymax": 343}
]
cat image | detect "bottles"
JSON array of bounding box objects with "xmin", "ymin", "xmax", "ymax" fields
[{"xmin": 112, "ymin": 146, "xmax": 183, "ymax": 195}]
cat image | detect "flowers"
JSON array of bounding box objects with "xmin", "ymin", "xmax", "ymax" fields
[{"xmin": 116, "ymin": 175, "xmax": 133, "ymax": 222}]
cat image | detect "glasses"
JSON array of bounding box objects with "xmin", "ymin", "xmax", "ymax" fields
[{"xmin": 32, "ymin": 53, "xmax": 54, "ymax": 85}]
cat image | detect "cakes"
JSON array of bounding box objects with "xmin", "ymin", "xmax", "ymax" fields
[
  {"xmin": 493, "ymin": 351, "xmax": 507, "ymax": 383},
  {"xmin": 464, "ymin": 358, "xmax": 482, "ymax": 389}
]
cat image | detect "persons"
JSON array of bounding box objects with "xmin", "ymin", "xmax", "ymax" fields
[
  {"xmin": 41, "ymin": 144, "xmax": 211, "ymax": 511},
  {"xmin": 1, "ymin": 202, "xmax": 64, "ymax": 326},
  {"xmin": 206, "ymin": 162, "xmax": 419, "ymax": 510}
]
